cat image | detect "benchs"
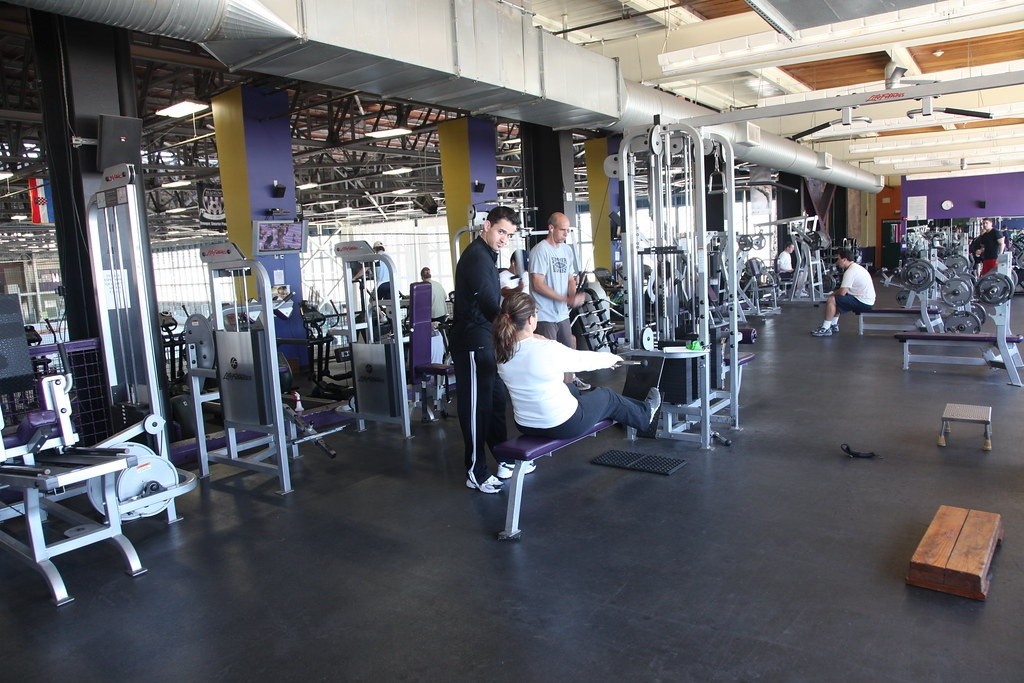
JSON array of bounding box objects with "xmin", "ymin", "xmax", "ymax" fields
[
  {"xmin": 408, "ymin": 281, "xmax": 434, "ymax": 386},
  {"xmin": 894, "ymin": 331, "xmax": 1024, "ymax": 387},
  {"xmin": 726, "ymin": 353, "xmax": 753, "ymax": 401},
  {"xmin": 171, "ymin": 409, "xmax": 357, "ymax": 475},
  {"xmin": 492, "ymin": 418, "xmax": 619, "ymax": 541},
  {"xmin": 858, "ymin": 309, "xmax": 943, "ymax": 336}
]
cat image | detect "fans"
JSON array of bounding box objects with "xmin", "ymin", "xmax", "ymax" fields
[
  {"xmin": 839, "ymin": 49, "xmax": 942, "ymax": 96},
  {"xmin": 942, "ymin": 158, "xmax": 991, "ymax": 169}
]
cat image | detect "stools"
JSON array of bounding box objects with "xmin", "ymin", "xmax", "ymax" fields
[
  {"xmin": 905, "ymin": 504, "xmax": 1004, "ymax": 601},
  {"xmin": 937, "ymin": 403, "xmax": 993, "ymax": 451}
]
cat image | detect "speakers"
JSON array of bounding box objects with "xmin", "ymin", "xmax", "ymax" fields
[{"xmin": 99, "ymin": 113, "xmax": 143, "ymax": 176}]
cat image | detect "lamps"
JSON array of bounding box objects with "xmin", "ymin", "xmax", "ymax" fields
[{"xmin": 155, "ymin": 97, "xmax": 415, "ymax": 206}]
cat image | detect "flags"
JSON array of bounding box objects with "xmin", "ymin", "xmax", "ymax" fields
[{"xmin": 27, "ymin": 177, "xmax": 55, "ymax": 224}]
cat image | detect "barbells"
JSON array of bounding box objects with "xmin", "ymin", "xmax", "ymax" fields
[
  {"xmin": 738, "ymin": 234, "xmax": 766, "ymax": 251},
  {"xmin": 805, "ymin": 230, "xmax": 832, "ymax": 251},
  {"xmin": 976, "ymin": 267, "xmax": 1019, "ymax": 306},
  {"xmin": 814, "ymin": 270, "xmax": 844, "ymax": 293},
  {"xmin": 896, "ymin": 253, "xmax": 987, "ymax": 334}
]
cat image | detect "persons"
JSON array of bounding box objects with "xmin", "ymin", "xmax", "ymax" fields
[
  {"xmin": 352, "ymin": 241, "xmax": 391, "ymax": 323},
  {"xmin": 450, "ymin": 206, "xmax": 537, "ymax": 495},
  {"xmin": 971, "ymin": 229, "xmax": 986, "ymax": 277},
  {"xmin": 809, "ymin": 250, "xmax": 876, "ymax": 337},
  {"xmin": 975, "ymin": 218, "xmax": 1004, "ymax": 276},
  {"xmin": 491, "ymin": 291, "xmax": 661, "ymax": 439},
  {"xmin": 777, "ymin": 242, "xmax": 811, "ymax": 298},
  {"xmin": 420, "ymin": 267, "xmax": 449, "ymax": 325},
  {"xmin": 528, "ymin": 212, "xmax": 591, "ymax": 391},
  {"xmin": 932, "ymin": 224, "xmax": 1024, "ymax": 251},
  {"xmin": 498, "ymin": 249, "xmax": 529, "ymax": 309}
]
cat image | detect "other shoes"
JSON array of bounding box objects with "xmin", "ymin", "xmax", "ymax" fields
[{"xmin": 794, "ymin": 290, "xmax": 809, "ymax": 297}]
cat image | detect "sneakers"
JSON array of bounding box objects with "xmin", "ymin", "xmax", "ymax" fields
[
  {"xmin": 497, "ymin": 459, "xmax": 535, "ymax": 478},
  {"xmin": 829, "ymin": 324, "xmax": 840, "ymax": 333},
  {"xmin": 572, "ymin": 377, "xmax": 591, "ymax": 391},
  {"xmin": 466, "ymin": 474, "xmax": 505, "ymax": 494},
  {"xmin": 647, "ymin": 387, "xmax": 661, "ymax": 424},
  {"xmin": 810, "ymin": 327, "xmax": 832, "ymax": 336}
]
